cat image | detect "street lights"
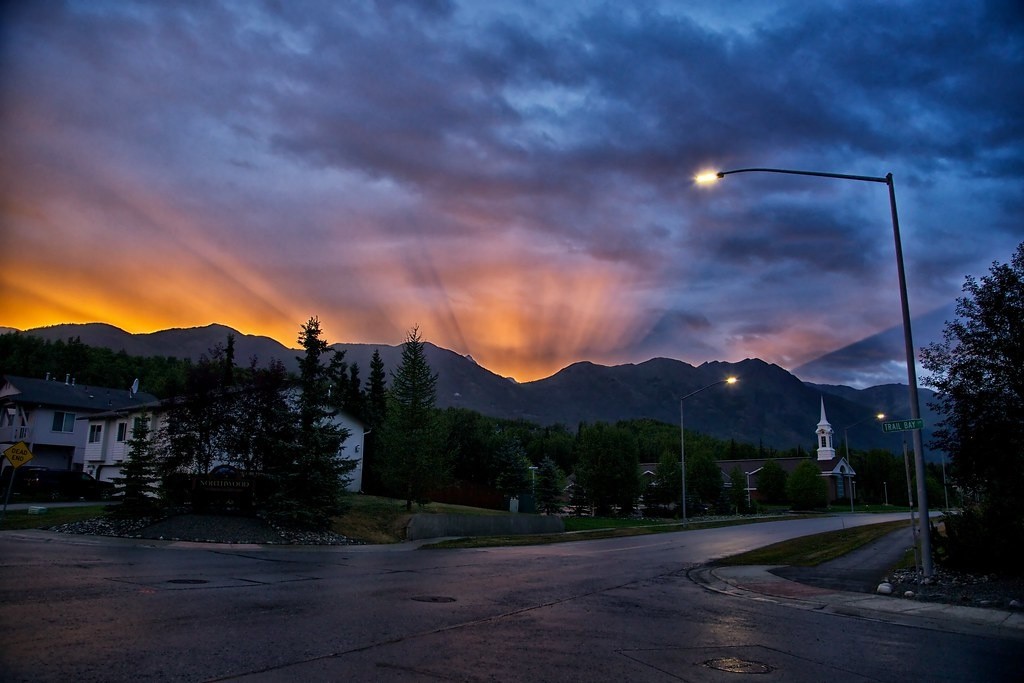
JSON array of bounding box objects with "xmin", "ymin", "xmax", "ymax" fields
[
  {"xmin": 845, "ymin": 414, "xmax": 885, "ymax": 512},
  {"xmin": 692, "ymin": 165, "xmax": 936, "ymax": 578},
  {"xmin": 679, "ymin": 376, "xmax": 736, "ymax": 525},
  {"xmin": 529, "ymin": 466, "xmax": 539, "ymax": 498}
]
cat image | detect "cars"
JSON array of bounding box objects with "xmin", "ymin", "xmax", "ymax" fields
[
  {"xmin": 0, "ymin": 465, "xmax": 50, "ymax": 504},
  {"xmin": 21, "ymin": 468, "xmax": 118, "ymax": 504}
]
